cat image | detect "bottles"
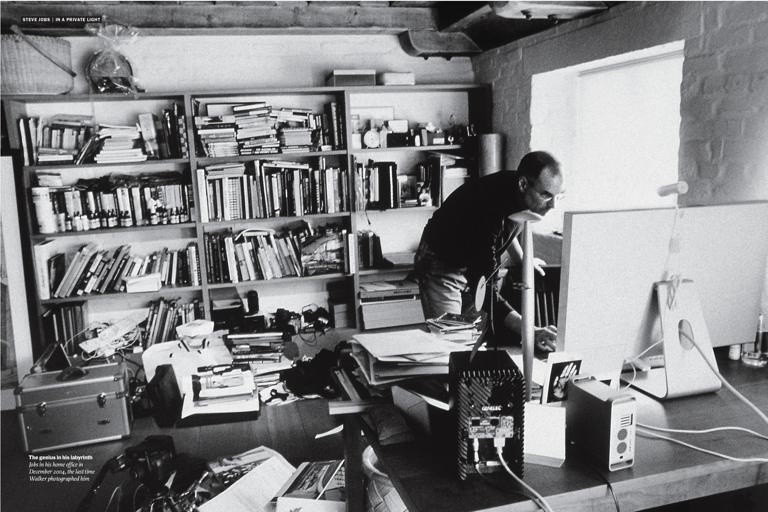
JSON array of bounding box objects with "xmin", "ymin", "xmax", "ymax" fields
[
  {"xmin": 64, "ymin": 206, "xmax": 189, "ymax": 232},
  {"xmin": 364, "ymin": 231, "xmax": 377, "ymax": 268}
]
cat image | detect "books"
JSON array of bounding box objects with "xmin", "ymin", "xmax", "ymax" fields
[{"xmin": 19, "ymin": 97, "xmax": 488, "ymax": 423}]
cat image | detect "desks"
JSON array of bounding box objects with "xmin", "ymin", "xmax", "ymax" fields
[{"xmin": 329, "ymin": 348, "xmax": 768, "ymax": 512}]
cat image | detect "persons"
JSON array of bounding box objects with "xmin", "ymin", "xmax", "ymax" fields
[
  {"xmin": 409, "ymin": 149, "xmax": 567, "ymax": 356},
  {"xmin": 551, "ymin": 362, "xmax": 578, "ymax": 400}
]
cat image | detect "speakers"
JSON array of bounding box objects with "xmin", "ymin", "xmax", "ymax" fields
[{"xmin": 565, "ymin": 374, "xmax": 638, "ymax": 472}]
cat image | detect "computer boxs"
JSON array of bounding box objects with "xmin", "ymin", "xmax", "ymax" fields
[{"xmin": 448, "ymin": 350, "xmax": 525, "ymax": 487}]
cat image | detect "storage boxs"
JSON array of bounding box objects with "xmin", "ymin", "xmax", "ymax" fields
[
  {"xmin": 378, "ymin": 72, "xmax": 416, "ymax": 86},
  {"xmin": 325, "ymin": 69, "xmax": 376, "ymax": 86},
  {"xmin": 13, "ymin": 362, "xmax": 134, "ymax": 456}
]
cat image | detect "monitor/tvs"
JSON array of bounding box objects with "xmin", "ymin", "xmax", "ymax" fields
[{"xmin": 554, "ymin": 199, "xmax": 768, "ymax": 402}]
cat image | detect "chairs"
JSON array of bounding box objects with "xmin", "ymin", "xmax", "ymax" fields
[{"xmin": 505, "ymin": 266, "xmax": 561, "ymax": 347}]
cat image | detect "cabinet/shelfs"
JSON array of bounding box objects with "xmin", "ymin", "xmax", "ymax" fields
[
  {"xmin": 347, "ymin": 82, "xmax": 492, "ymax": 333},
  {"xmin": 189, "ymin": 86, "xmax": 356, "ymax": 346},
  {"xmin": 0, "ymin": 92, "xmax": 209, "ymax": 369}
]
